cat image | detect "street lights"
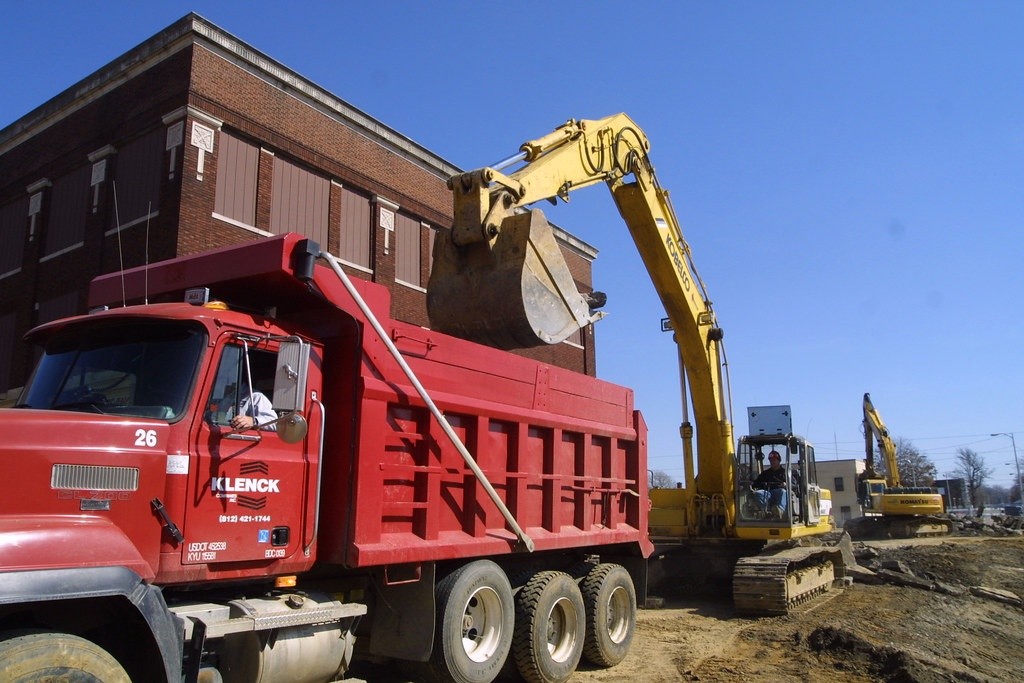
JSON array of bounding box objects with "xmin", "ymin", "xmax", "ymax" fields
[
  {"xmin": 945, "ymin": 471, "xmax": 956, "ymax": 509},
  {"xmin": 913, "ymin": 455, "xmax": 927, "ymax": 486},
  {"xmin": 991, "ymin": 432, "xmax": 1024, "ymax": 504}
]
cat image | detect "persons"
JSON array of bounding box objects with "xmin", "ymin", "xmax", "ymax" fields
[
  {"xmin": 204, "ymin": 353, "xmax": 280, "ymax": 431},
  {"xmin": 748, "ymin": 450, "xmax": 800, "ymax": 522}
]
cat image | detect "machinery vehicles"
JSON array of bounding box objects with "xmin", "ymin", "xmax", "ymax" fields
[
  {"xmin": 844, "ymin": 392, "xmax": 953, "ymax": 540},
  {"xmin": 425, "ymin": 111, "xmax": 847, "ymax": 618},
  {"xmin": 0, "ymin": 233, "xmax": 654, "ymax": 683}
]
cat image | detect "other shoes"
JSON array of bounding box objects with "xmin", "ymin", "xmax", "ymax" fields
[{"xmin": 772, "ymin": 506, "xmax": 781, "ymax": 519}]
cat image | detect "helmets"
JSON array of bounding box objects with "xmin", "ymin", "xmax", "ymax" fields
[{"xmin": 768, "ymin": 451, "xmax": 781, "ymax": 461}]
